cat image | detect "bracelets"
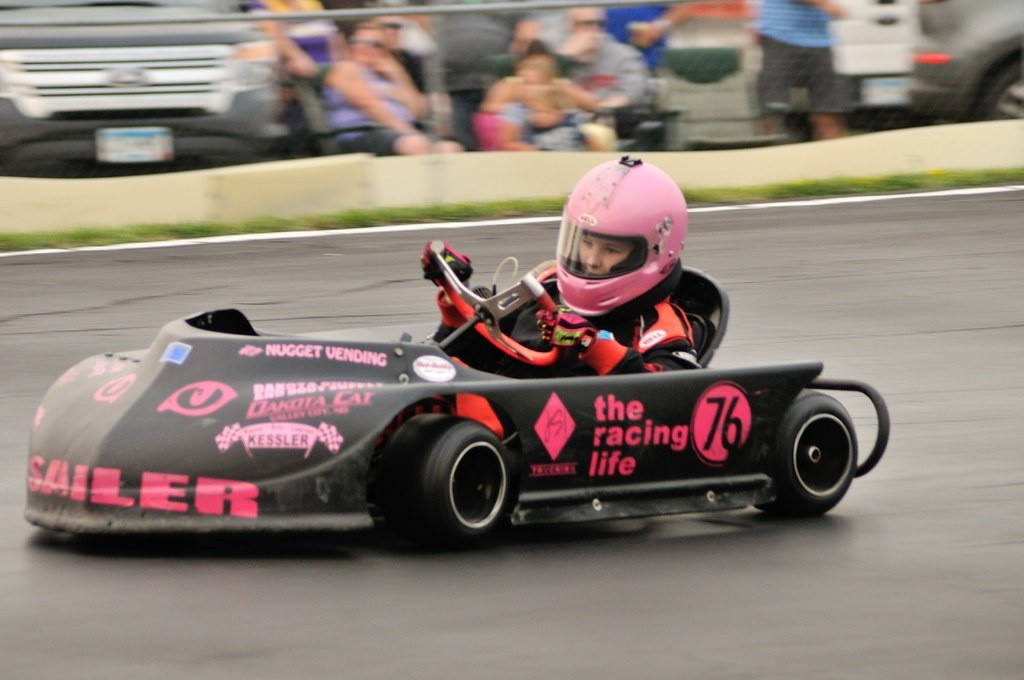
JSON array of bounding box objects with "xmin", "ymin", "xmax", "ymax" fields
[{"xmin": 653, "ymin": 15, "xmax": 674, "ymax": 33}]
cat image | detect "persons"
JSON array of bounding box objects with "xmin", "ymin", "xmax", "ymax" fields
[
  {"xmin": 747, "ymin": 0, "xmax": 853, "ymax": 147},
  {"xmin": 419, "ymin": 159, "xmax": 703, "ymax": 376},
  {"xmin": 240, "ymin": 0, "xmax": 694, "ymax": 152},
  {"xmin": 477, "ymin": 52, "xmax": 616, "ymax": 151}
]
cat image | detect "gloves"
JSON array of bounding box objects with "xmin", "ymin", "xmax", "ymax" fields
[
  {"xmin": 536, "ymin": 310, "xmax": 598, "ymax": 352},
  {"xmin": 420, "ymin": 238, "xmax": 475, "ymax": 289}
]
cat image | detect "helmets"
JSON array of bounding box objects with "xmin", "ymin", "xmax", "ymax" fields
[{"xmin": 556, "ymin": 157, "xmax": 687, "ymax": 320}]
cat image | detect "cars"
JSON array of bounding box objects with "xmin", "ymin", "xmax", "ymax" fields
[
  {"xmin": 905, "ymin": 1, "xmax": 1024, "ymax": 125},
  {"xmin": 2, "ymin": 1, "xmax": 289, "ymax": 179},
  {"xmin": 790, "ymin": 0, "xmax": 913, "ymax": 133}
]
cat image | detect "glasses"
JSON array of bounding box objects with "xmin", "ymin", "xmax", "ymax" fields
[
  {"xmin": 371, "ymin": 20, "xmax": 401, "ymax": 29},
  {"xmin": 577, "ymin": 18, "xmax": 607, "ymax": 27},
  {"xmin": 349, "ymin": 37, "xmax": 384, "ymax": 48}
]
